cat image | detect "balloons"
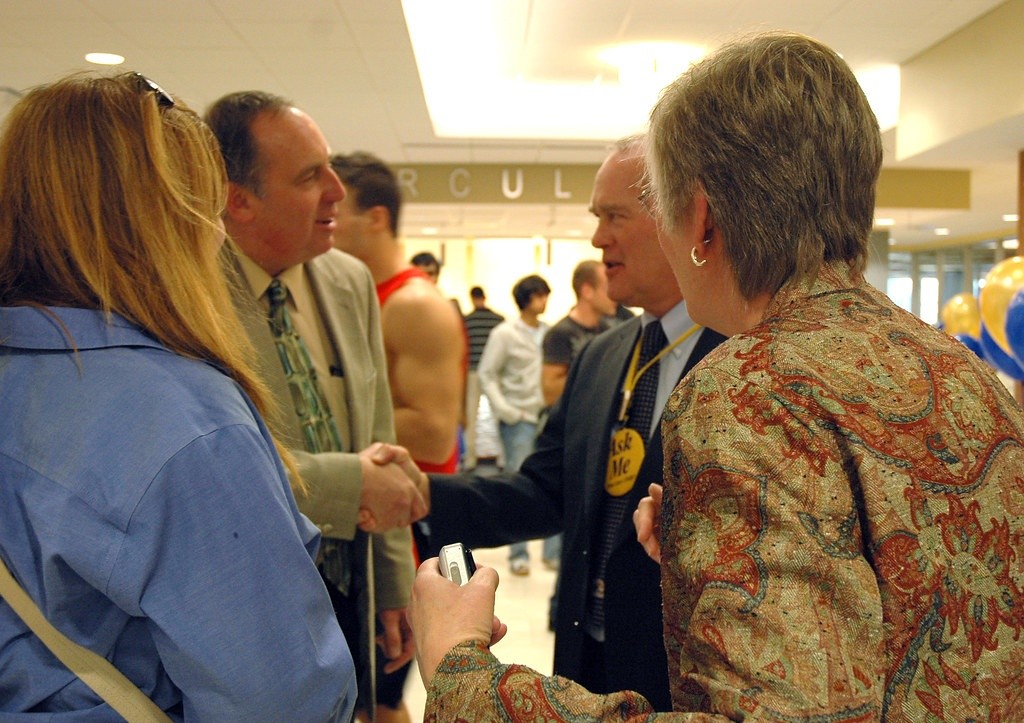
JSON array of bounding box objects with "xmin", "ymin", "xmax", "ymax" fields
[{"xmin": 941, "ymin": 294, "xmax": 995, "ymax": 373}]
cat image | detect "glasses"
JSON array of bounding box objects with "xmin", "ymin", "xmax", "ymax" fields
[{"xmin": 130, "ymin": 71, "xmax": 175, "ymax": 106}]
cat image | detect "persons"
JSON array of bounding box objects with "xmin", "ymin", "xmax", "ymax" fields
[
  {"xmin": 406, "ymin": 36, "xmax": 1024, "ymax": 723},
  {"xmin": 0, "ymin": 72, "xmax": 358, "ymax": 723},
  {"xmin": 329, "ymin": 151, "xmax": 638, "ymax": 580},
  {"xmin": 358, "ymin": 138, "xmax": 726, "ymax": 714},
  {"xmin": 203, "ymin": 92, "xmax": 429, "ymax": 723}
]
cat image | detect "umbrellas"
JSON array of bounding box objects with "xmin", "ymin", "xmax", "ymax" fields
[{"xmin": 980, "ymin": 255, "xmax": 1024, "ymax": 381}]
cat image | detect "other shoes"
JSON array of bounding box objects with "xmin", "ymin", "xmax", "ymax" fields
[
  {"xmin": 511, "ymin": 559, "xmax": 529, "ymax": 575},
  {"xmin": 544, "ymin": 559, "xmax": 561, "ymax": 570}
]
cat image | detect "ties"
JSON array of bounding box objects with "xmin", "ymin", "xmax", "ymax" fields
[
  {"xmin": 589, "ymin": 321, "xmax": 668, "ymax": 642},
  {"xmin": 267, "ymin": 279, "xmax": 353, "ymax": 596}
]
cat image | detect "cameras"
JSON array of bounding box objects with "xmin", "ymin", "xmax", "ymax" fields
[{"xmin": 438, "ymin": 543, "xmax": 472, "ymax": 586}]
